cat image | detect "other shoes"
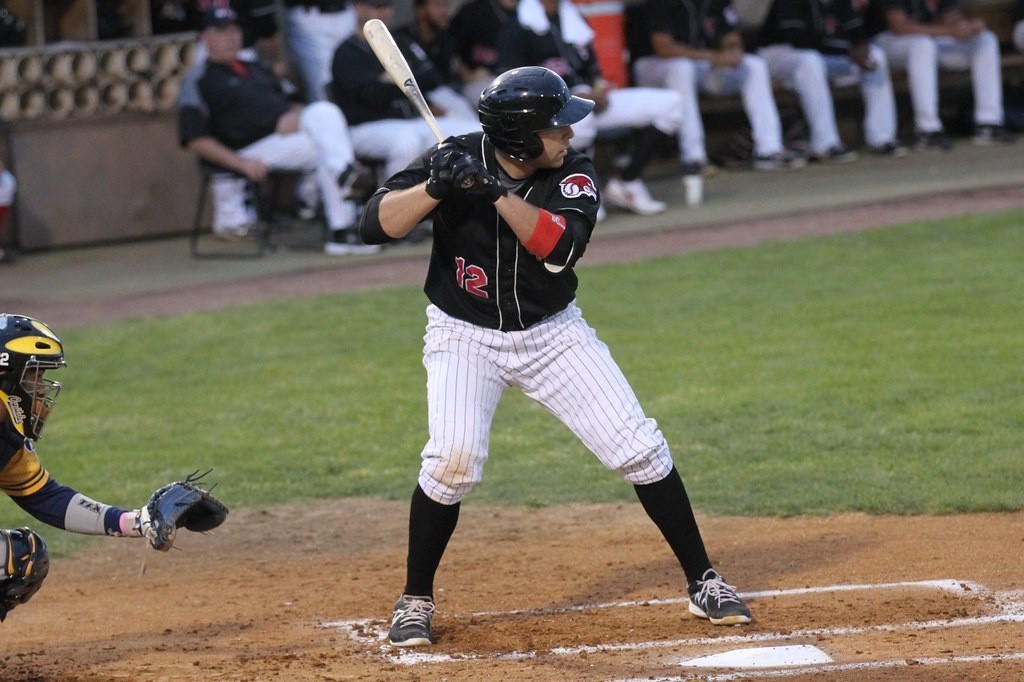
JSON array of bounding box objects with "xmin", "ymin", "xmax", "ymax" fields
[
  {"xmin": 809, "ymin": 145, "xmax": 860, "ymax": 164},
  {"xmin": 970, "ymin": 126, "xmax": 1017, "ymax": 146},
  {"xmin": 913, "ymin": 131, "xmax": 953, "ymax": 152},
  {"xmin": 755, "ymin": 154, "xmax": 808, "ymax": 173},
  {"xmin": 325, "ymin": 230, "xmax": 382, "ymax": 255},
  {"xmin": 873, "ymin": 141, "xmax": 912, "ymax": 159},
  {"xmin": 681, "ymin": 162, "xmax": 726, "ymax": 183},
  {"xmin": 605, "ymin": 176, "xmax": 664, "ymax": 215}
]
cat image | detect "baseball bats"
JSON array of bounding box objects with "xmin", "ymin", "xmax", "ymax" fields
[{"xmin": 362, "ymin": 18, "xmax": 474, "ymax": 190}]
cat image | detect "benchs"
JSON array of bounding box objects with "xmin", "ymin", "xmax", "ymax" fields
[{"xmin": 698, "ymin": 0, "xmax": 1024, "ymax": 170}]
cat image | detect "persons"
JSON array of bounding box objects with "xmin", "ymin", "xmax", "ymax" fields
[
  {"xmin": 0, "ymin": 315, "xmax": 229, "ymax": 622},
  {"xmin": 279, "ymin": 0, "xmax": 1024, "ymax": 227},
  {"xmin": 0, "ymin": 163, "xmax": 17, "ymax": 260},
  {"xmin": 179, "ymin": 0, "xmax": 380, "ymax": 254},
  {"xmin": 359, "ymin": 67, "xmax": 754, "ymax": 648}
]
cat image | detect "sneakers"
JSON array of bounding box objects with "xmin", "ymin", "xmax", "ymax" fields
[
  {"xmin": 389, "ymin": 592, "xmax": 435, "ymax": 647},
  {"xmin": 686, "ymin": 574, "xmax": 752, "ymax": 625}
]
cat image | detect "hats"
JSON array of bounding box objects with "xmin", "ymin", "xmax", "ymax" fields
[{"xmin": 200, "ymin": 7, "xmax": 245, "ymax": 28}]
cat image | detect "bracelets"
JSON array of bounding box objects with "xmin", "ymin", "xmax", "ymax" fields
[{"xmin": 120, "ymin": 513, "xmax": 128, "ymax": 536}]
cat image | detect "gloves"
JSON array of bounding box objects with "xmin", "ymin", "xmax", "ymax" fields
[{"xmin": 426, "ymin": 136, "xmax": 506, "ymax": 206}]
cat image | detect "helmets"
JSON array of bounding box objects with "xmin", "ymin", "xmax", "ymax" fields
[
  {"xmin": 0, "ymin": 312, "xmax": 66, "ymax": 441},
  {"xmin": 478, "ymin": 66, "xmax": 596, "ymax": 162}
]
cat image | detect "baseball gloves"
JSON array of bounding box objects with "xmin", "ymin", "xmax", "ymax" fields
[{"xmin": 140, "ymin": 480, "xmax": 233, "ymax": 555}]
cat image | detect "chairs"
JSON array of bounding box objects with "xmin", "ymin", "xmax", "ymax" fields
[{"xmin": 189, "ymin": 154, "xmax": 303, "ymax": 261}]
